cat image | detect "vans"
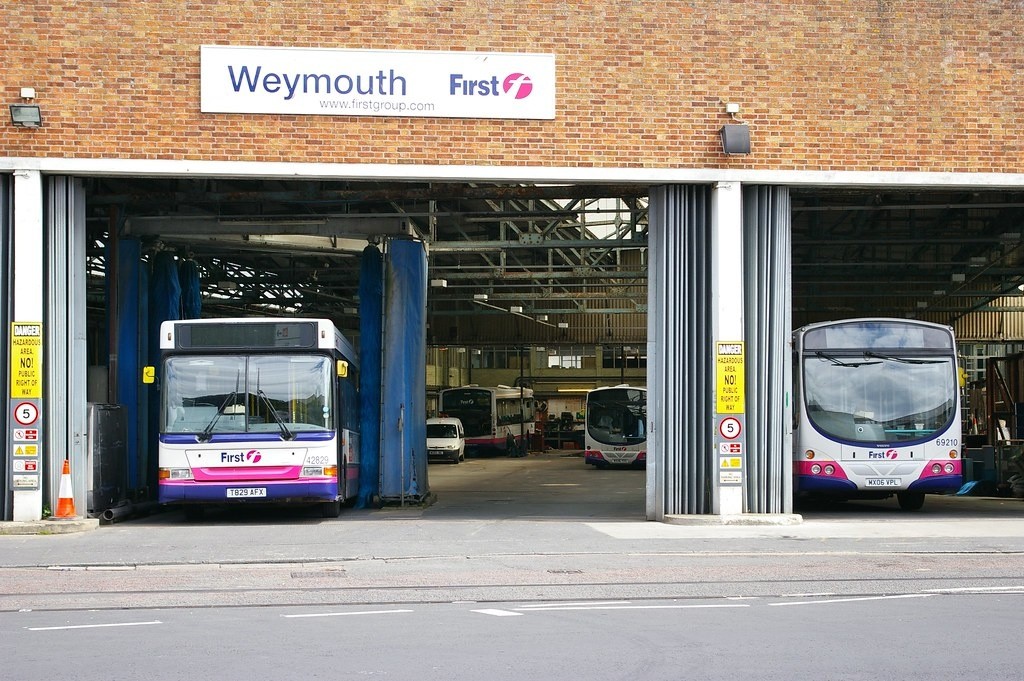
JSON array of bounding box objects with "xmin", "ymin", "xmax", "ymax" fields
[{"xmin": 425, "ymin": 416, "xmax": 466, "ymax": 465}]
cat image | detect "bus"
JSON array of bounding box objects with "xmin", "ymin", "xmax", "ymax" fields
[
  {"xmin": 584, "ymin": 383, "xmax": 648, "ymax": 469},
  {"xmin": 440, "ymin": 382, "xmax": 538, "ymax": 456},
  {"xmin": 143, "ymin": 318, "xmax": 361, "ymax": 517},
  {"xmin": 791, "ymin": 317, "xmax": 969, "ymax": 510}
]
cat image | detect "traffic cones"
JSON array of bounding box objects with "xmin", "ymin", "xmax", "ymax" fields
[{"xmin": 49, "ymin": 459, "xmax": 85, "ymax": 521}]
